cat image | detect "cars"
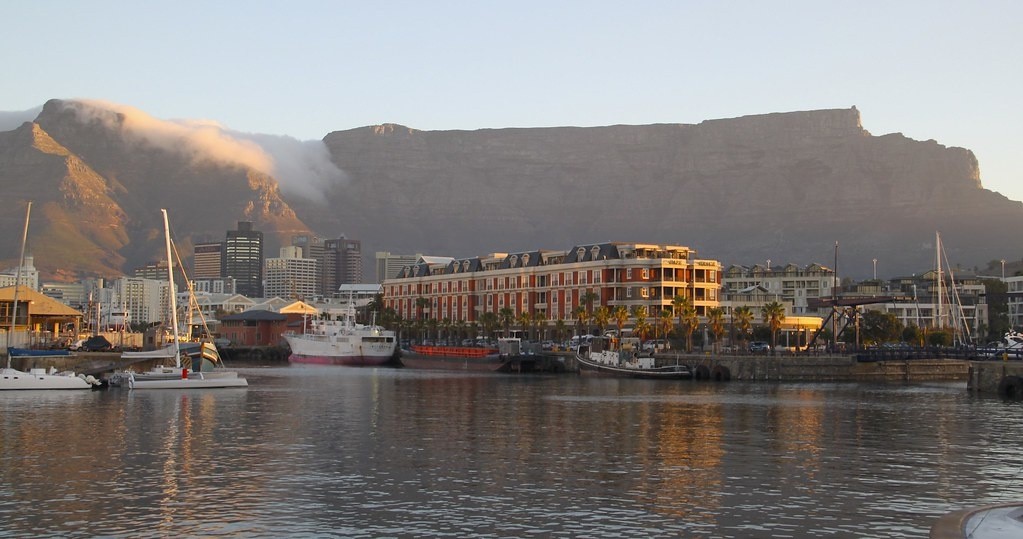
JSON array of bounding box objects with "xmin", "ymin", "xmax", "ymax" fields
[
  {"xmin": 68, "ymin": 338, "xmax": 88, "ymax": 352},
  {"xmin": 748, "ymin": 341, "xmax": 771, "ymax": 355},
  {"xmin": 425, "ymin": 336, "xmax": 497, "ymax": 348},
  {"xmin": 522, "ymin": 339, "xmax": 580, "ymax": 352}
]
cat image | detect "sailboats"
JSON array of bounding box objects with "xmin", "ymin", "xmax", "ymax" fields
[
  {"xmin": 911, "ymin": 228, "xmax": 1023, "ymax": 361},
  {"xmin": 100, "ymin": 208, "xmax": 249, "ymax": 392},
  {"xmin": 0, "ymin": 201, "xmax": 102, "ymax": 392}
]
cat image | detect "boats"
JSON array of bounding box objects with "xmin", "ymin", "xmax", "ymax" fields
[
  {"xmin": 575, "ymin": 335, "xmax": 696, "ymax": 381},
  {"xmin": 280, "ymin": 286, "xmax": 399, "ymax": 366},
  {"xmin": 393, "ymin": 334, "xmax": 539, "ymax": 374}
]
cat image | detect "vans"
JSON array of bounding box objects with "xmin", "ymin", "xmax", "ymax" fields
[{"xmin": 642, "ymin": 339, "xmax": 671, "ymax": 353}]
